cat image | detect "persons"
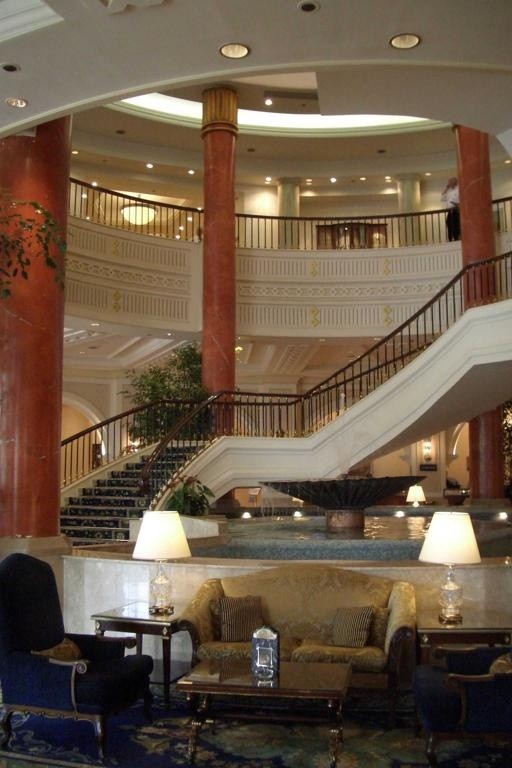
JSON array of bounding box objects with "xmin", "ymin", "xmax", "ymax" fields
[{"xmin": 439, "ymin": 175, "xmax": 460, "ymax": 242}]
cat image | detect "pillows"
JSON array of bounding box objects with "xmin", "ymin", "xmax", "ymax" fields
[
  {"xmin": 219, "ymin": 595, "xmax": 262, "ymax": 642},
  {"xmin": 490, "ymin": 651, "xmax": 512, "ymax": 674},
  {"xmin": 31, "ymin": 638, "xmax": 81, "ymax": 661},
  {"xmin": 325, "ymin": 606, "xmax": 375, "ymax": 648},
  {"xmin": 209, "ymin": 598, "xmax": 222, "ymax": 642},
  {"xmin": 367, "ymin": 608, "xmax": 392, "ymax": 648}
]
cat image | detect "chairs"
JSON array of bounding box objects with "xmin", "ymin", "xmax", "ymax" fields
[
  {"xmin": 0, "ymin": 553, "xmax": 155, "ymax": 768},
  {"xmin": 425, "ymin": 645, "xmax": 511, "ymax": 768}
]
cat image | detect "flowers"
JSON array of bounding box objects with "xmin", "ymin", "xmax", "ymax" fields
[{"xmin": 164, "ymin": 474, "xmax": 214, "ymax": 516}]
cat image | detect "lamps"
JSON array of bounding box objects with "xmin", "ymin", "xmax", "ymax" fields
[
  {"xmin": 418, "ymin": 511, "xmax": 482, "ymax": 625},
  {"xmin": 406, "ymin": 484, "xmax": 426, "ymax": 507},
  {"xmin": 132, "ymin": 510, "xmax": 192, "ymax": 613},
  {"xmin": 121, "ymin": 204, "xmax": 158, "ymax": 226}
]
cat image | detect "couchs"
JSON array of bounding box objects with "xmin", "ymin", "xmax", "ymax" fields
[{"xmin": 177, "ymin": 564, "xmax": 418, "ymax": 725}]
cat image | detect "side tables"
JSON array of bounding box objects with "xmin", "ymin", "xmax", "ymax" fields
[
  {"xmin": 90, "ymin": 601, "xmax": 192, "ymax": 699},
  {"xmin": 417, "ymin": 615, "xmax": 511, "ymax": 667}
]
